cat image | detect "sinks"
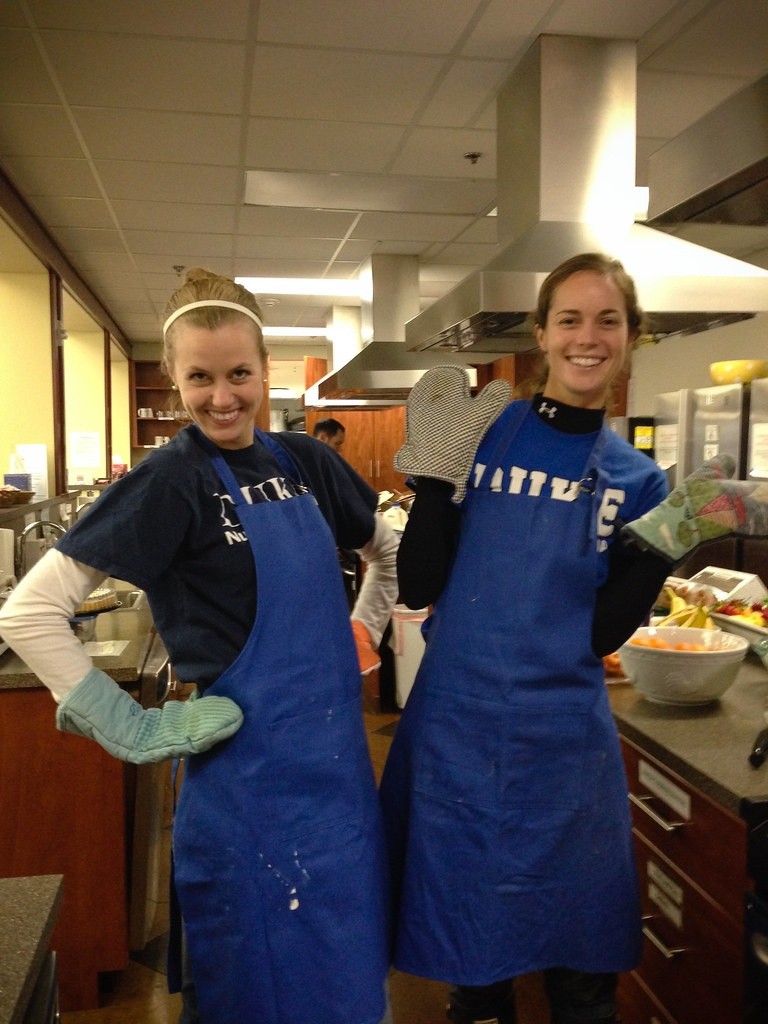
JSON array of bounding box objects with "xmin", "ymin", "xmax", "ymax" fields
[{"xmin": 108, "ymin": 588, "xmax": 139, "ymax": 609}]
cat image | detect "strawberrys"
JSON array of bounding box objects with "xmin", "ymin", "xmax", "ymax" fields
[{"xmin": 717, "ymin": 599, "xmax": 768, "ymax": 619}]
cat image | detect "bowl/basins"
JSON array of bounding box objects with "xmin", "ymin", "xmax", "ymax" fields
[
  {"xmin": 709, "ymin": 360, "xmax": 768, "ymax": 385},
  {"xmin": 620, "ymin": 626, "xmax": 750, "ymax": 707}
]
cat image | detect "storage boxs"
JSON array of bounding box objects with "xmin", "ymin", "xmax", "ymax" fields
[{"xmin": 677, "ymin": 566, "xmax": 768, "ymax": 607}]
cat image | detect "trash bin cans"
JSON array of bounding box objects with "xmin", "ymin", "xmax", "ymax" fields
[{"xmin": 390, "ymin": 603, "xmax": 431, "ymax": 709}]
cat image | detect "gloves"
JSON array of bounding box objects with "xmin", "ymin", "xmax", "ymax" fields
[
  {"xmin": 56, "ymin": 666, "xmax": 243, "ymax": 765},
  {"xmin": 393, "ymin": 365, "xmax": 513, "ymax": 504},
  {"xmin": 621, "ymin": 453, "xmax": 768, "ymax": 568},
  {"xmin": 351, "ymin": 619, "xmax": 381, "ymax": 676}
]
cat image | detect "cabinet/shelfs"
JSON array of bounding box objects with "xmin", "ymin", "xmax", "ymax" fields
[
  {"xmin": 0, "ymin": 631, "xmax": 180, "ymax": 1014},
  {"xmin": 617, "ymin": 734, "xmax": 767, "ymax": 1024},
  {"xmin": 306, "ymin": 405, "xmax": 410, "ymax": 492},
  {"xmin": 129, "ymin": 359, "xmax": 194, "ymax": 448},
  {"xmin": 255, "ymin": 354, "xmax": 328, "ymax": 433}
]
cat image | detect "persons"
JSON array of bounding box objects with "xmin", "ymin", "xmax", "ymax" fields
[
  {"xmin": 1, "ymin": 270, "xmax": 399, "ymax": 1024},
  {"xmin": 380, "ymin": 254, "xmax": 767, "ymax": 1024}
]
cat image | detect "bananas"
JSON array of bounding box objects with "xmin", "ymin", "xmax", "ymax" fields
[{"xmin": 656, "ymin": 587, "xmax": 716, "ymax": 631}]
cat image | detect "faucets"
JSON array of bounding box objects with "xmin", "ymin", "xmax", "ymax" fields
[
  {"xmin": 75, "ymin": 501, "xmax": 94, "ymax": 519},
  {"xmin": 17, "ymin": 520, "xmax": 66, "ymax": 582}
]
